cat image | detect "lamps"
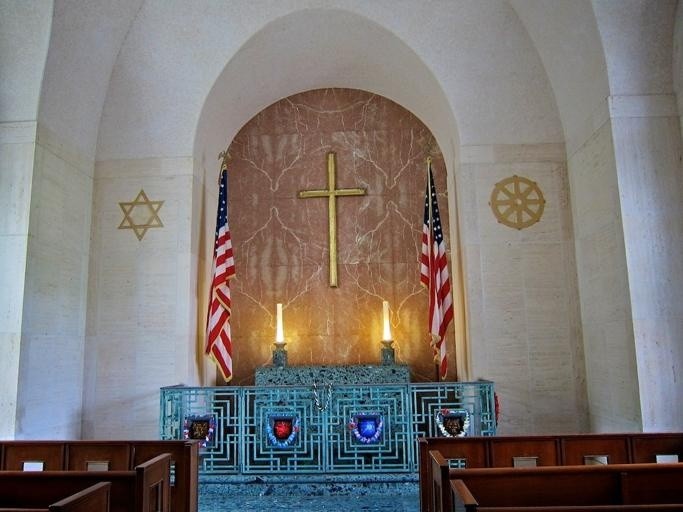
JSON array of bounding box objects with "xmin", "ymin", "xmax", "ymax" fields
[
  {"xmin": 379, "ymin": 300, "xmax": 395, "ymax": 364},
  {"xmin": 272, "ymin": 302, "xmax": 287, "ymax": 366}
]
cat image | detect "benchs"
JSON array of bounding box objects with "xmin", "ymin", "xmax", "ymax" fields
[
  {"xmin": 417, "ymin": 431, "xmax": 683, "ymax": 512},
  {"xmin": 0, "ymin": 440, "xmax": 200, "ymax": 512}
]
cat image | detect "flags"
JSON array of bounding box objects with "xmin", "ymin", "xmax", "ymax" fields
[
  {"xmin": 204, "ymin": 163, "xmax": 236, "ymax": 384},
  {"xmin": 417, "ymin": 163, "xmax": 454, "ymax": 380}
]
